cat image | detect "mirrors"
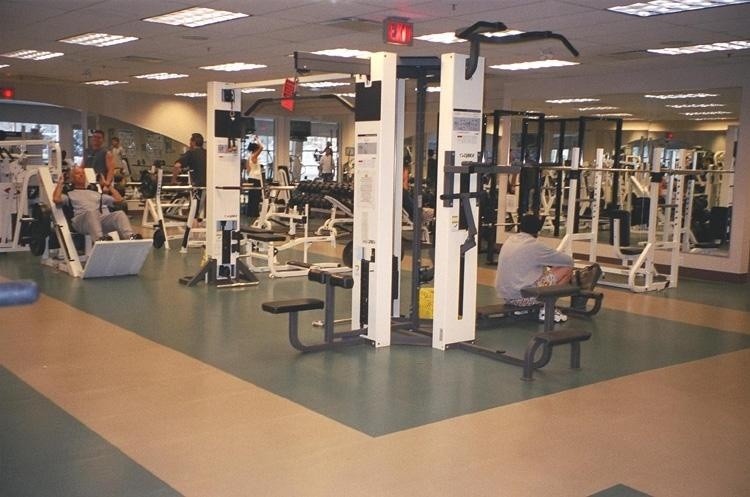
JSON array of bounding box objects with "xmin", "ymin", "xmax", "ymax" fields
[{"xmin": 502, "ymin": 85, "xmax": 744, "ymax": 259}]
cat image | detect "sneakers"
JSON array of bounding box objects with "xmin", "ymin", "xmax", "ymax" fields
[
  {"xmin": 539, "ymin": 307, "xmax": 568, "ymax": 323},
  {"xmin": 129, "ymin": 234, "xmax": 142, "ymax": 239},
  {"xmin": 514, "ymin": 310, "xmax": 532, "ymax": 314},
  {"xmin": 91, "ymin": 236, "xmax": 113, "ymax": 247}
]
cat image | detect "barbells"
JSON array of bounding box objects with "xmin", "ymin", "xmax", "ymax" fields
[{"xmin": 137, "ymin": 159, "xmax": 146, "ymax": 165}]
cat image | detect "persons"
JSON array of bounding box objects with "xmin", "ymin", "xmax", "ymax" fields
[
  {"xmin": 402, "ymin": 166, "xmax": 413, "ymax": 218},
  {"xmin": 245, "ymin": 136, "xmax": 264, "ymax": 225},
  {"xmin": 672, "ymin": 178, "xmax": 708, "ymax": 219},
  {"xmin": 260, "ymin": 164, "xmax": 265, "ymax": 176},
  {"xmin": 427, "ymin": 149, "xmax": 437, "ymax": 179},
  {"xmin": 648, "ymin": 178, "xmax": 665, "ymax": 215},
  {"xmin": 319, "ymin": 147, "xmax": 336, "ymax": 182},
  {"xmin": 170, "ymin": 132, "xmax": 207, "ymax": 225},
  {"xmin": 52, "ymin": 130, "xmax": 143, "ymax": 249},
  {"xmin": 494, "ymin": 214, "xmax": 575, "ymax": 324}
]
